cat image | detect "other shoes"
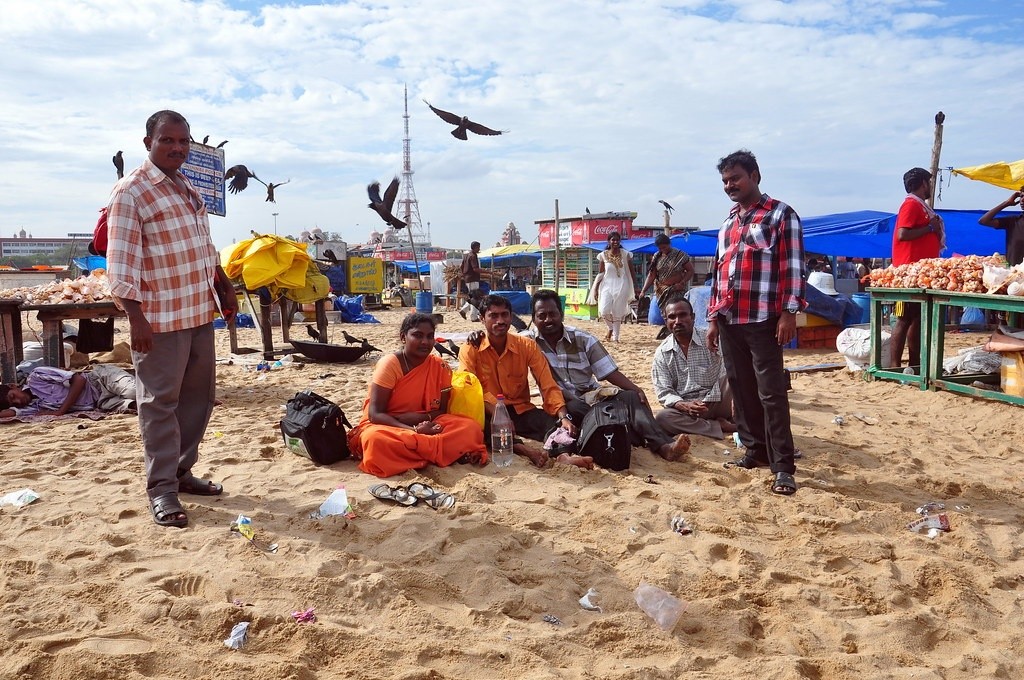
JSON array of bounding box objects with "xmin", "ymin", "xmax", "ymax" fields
[
  {"xmin": 471, "ymin": 317, "xmax": 481, "ymax": 322},
  {"xmin": 458, "ymin": 310, "xmax": 467, "ymax": 320}
]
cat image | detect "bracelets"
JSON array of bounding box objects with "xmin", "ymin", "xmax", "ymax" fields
[
  {"xmin": 413, "ymin": 424, "xmax": 418, "ymax": 433},
  {"xmin": 428, "ymin": 413, "xmax": 432, "ymax": 422},
  {"xmin": 928, "ymin": 224, "xmax": 933, "ymax": 233}
]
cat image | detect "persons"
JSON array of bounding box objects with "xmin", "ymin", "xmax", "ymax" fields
[
  {"xmin": 891, "ymin": 166, "xmax": 941, "ymax": 367},
  {"xmin": 106, "ymin": 111, "xmax": 238, "ymax": 525},
  {"xmin": 653, "ymin": 295, "xmax": 738, "ymax": 440},
  {"xmin": 459, "ymin": 242, "xmax": 492, "ymax": 322},
  {"xmin": 705, "ymin": 151, "xmax": 810, "ymax": 494},
  {"xmin": 705, "ymin": 257, "xmax": 872, "ymax": 296},
  {"xmin": 640, "ymin": 234, "xmax": 695, "ymax": 324},
  {"xmin": 502, "ymin": 269, "xmax": 541, "ymax": 287},
  {"xmin": 0, "ymin": 366, "xmax": 138, "ymax": 417},
  {"xmin": 593, "ymin": 232, "xmax": 639, "ymax": 341},
  {"xmin": 348, "ymin": 313, "xmax": 488, "ymax": 477},
  {"xmin": 458, "ymin": 290, "xmax": 691, "ymax": 470},
  {"xmin": 978, "ymin": 186, "xmax": 1024, "ymax": 328}
]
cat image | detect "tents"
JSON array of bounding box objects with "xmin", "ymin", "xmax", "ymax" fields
[
  {"xmin": 393, "ymin": 260, "xmax": 430, "ymax": 284},
  {"xmin": 70, "ymin": 255, "xmax": 107, "ymax": 281},
  {"xmin": 583, "ymin": 210, "xmax": 1021, "ymax": 291},
  {"xmin": 479, "ymin": 245, "xmax": 542, "ymax": 286}
]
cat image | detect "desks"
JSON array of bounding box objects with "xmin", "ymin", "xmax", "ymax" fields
[
  {"xmin": 489, "ymin": 290, "xmax": 531, "ymax": 314},
  {"xmin": 864, "ymin": 286, "xmax": 1024, "ymax": 407},
  {"xmin": 0, "ymin": 298, "xmax": 129, "ymax": 384}
]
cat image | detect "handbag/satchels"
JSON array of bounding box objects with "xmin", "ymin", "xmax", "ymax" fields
[
  {"xmin": 279, "ymin": 391, "xmax": 353, "ymax": 465},
  {"xmin": 573, "ymin": 397, "xmax": 635, "ymax": 472}
]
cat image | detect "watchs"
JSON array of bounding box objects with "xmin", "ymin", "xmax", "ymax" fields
[
  {"xmin": 783, "ymin": 309, "xmax": 798, "ymax": 314},
  {"xmin": 556, "ymin": 414, "xmax": 574, "ymax": 424}
]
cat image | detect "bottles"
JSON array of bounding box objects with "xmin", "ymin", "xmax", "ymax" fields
[
  {"xmin": 490, "ymin": 394, "xmax": 514, "ymax": 468},
  {"xmin": 899, "ymin": 367, "xmax": 914, "ymax": 385},
  {"xmin": 271, "ymin": 354, "xmax": 294, "ymax": 371}
]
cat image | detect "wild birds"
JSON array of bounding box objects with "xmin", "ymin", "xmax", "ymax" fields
[
  {"xmin": 305, "ymin": 324, "xmax": 320, "ymax": 343},
  {"xmin": 252, "ymin": 171, "xmax": 290, "ymax": 202},
  {"xmin": 368, "ymin": 174, "xmax": 408, "ymax": 229},
  {"xmin": 423, "ymin": 98, "xmax": 510, "ymax": 140},
  {"xmin": 585, "ymin": 207, "xmax": 591, "ymax": 214},
  {"xmin": 113, "ymin": 150, "xmax": 124, "ymax": 177},
  {"xmin": 224, "ymin": 165, "xmax": 255, "ymax": 194},
  {"xmin": 362, "ymin": 337, "xmax": 382, "ymax": 360},
  {"xmin": 203, "ymin": 135, "xmax": 209, "ymax": 145},
  {"xmin": 342, "ymin": 330, "xmax": 363, "ymax": 346},
  {"xmin": 659, "ymin": 199, "xmax": 675, "ymax": 215},
  {"xmin": 934, "ymin": 110, "xmax": 946, "ymax": 125},
  {"xmin": 434, "ymin": 337, "xmax": 461, "ymax": 359}
]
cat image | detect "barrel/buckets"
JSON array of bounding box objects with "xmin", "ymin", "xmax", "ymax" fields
[
  {"xmin": 416, "ymin": 292, "xmax": 433, "ymax": 314},
  {"xmin": 851, "ymin": 293, "xmax": 870, "ymax": 323},
  {"xmin": 648, "ymin": 296, "xmax": 665, "ymax": 325}
]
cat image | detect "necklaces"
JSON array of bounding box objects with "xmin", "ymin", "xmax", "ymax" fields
[{"xmin": 403, "ymin": 350, "xmax": 410, "ymax": 372}]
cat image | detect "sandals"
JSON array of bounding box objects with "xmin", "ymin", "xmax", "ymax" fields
[
  {"xmin": 770, "ymin": 471, "xmax": 797, "ymax": 496},
  {"xmin": 178, "ymin": 474, "xmax": 223, "ymax": 495},
  {"xmin": 722, "ymin": 458, "xmax": 770, "ymax": 470},
  {"xmin": 367, "ymin": 483, "xmax": 419, "ymax": 507},
  {"xmin": 407, "ymin": 481, "xmax": 455, "ymax": 512},
  {"xmin": 150, "ymin": 492, "xmax": 188, "ymax": 526}
]
